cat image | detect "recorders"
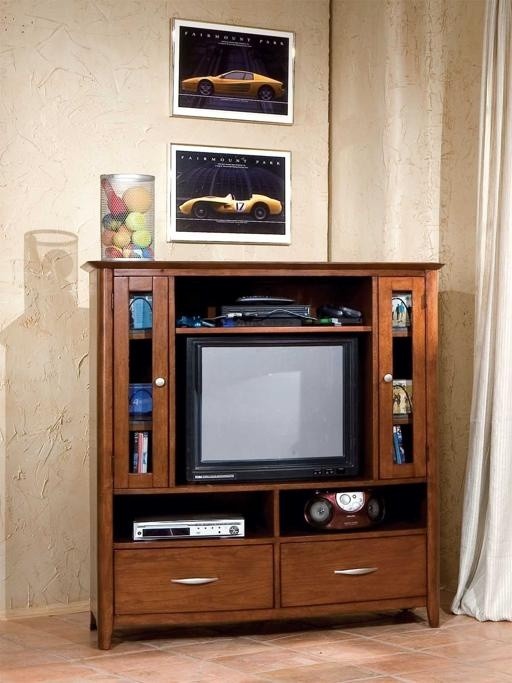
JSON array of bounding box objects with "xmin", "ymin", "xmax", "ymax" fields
[{"xmin": 302, "ymin": 488, "xmax": 386, "ymax": 531}]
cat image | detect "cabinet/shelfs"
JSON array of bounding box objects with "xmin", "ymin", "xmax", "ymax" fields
[{"xmin": 88, "ymin": 259, "xmax": 447, "ymax": 650}]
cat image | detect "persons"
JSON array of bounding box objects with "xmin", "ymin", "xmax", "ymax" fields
[{"xmin": 391, "ymin": 297, "xmax": 406, "ymax": 322}]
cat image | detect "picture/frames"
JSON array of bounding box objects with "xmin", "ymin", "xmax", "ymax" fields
[{"xmin": 170, "ymin": 16, "xmax": 294, "ymax": 245}]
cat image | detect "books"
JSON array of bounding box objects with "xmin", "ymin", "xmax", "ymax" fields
[
  {"xmin": 392, "ymin": 425, "xmax": 405, "ymax": 465},
  {"xmin": 133, "ymin": 431, "xmax": 149, "ymax": 473}
]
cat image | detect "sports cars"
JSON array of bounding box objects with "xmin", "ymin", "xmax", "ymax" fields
[
  {"xmin": 179, "ymin": 193, "xmax": 282, "ymax": 221},
  {"xmin": 181, "ymin": 70, "xmax": 285, "ymax": 101}
]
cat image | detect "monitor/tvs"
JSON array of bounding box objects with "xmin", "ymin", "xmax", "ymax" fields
[{"xmin": 185, "ymin": 337, "xmax": 359, "ymax": 481}]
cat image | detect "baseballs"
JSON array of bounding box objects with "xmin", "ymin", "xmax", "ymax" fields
[{"xmin": 101, "ymin": 186, "xmax": 154, "ymax": 257}]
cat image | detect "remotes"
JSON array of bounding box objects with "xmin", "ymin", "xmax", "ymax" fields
[{"xmin": 236, "ymin": 295, "xmax": 297, "ymax": 305}]
